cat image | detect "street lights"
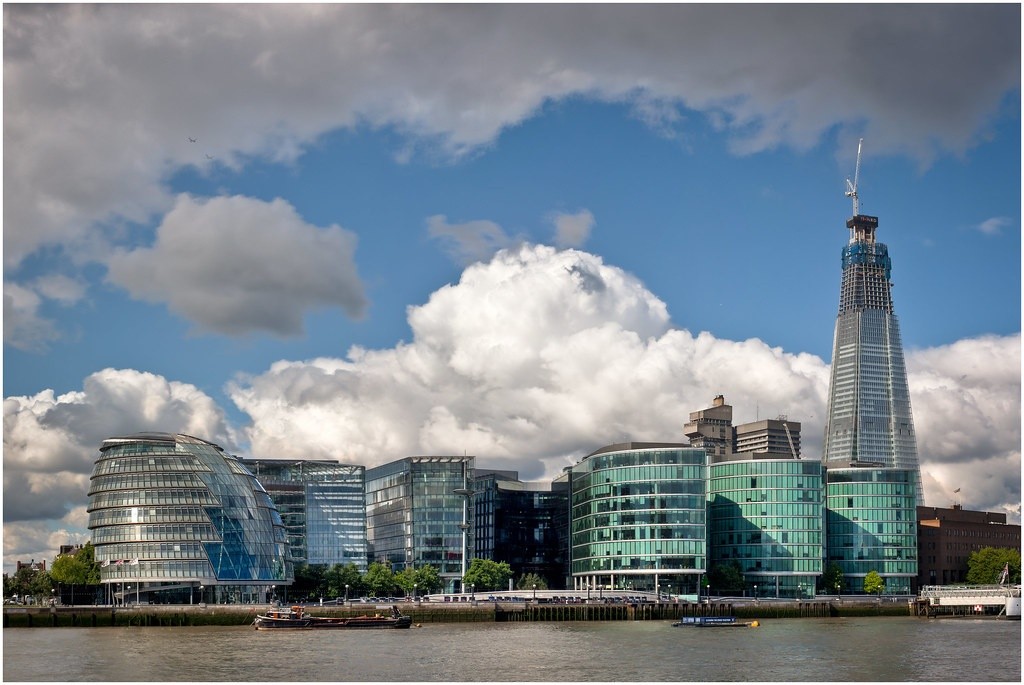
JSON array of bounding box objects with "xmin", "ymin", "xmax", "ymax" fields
[
  {"xmin": 126, "ymin": 585, "xmax": 131, "ymax": 607},
  {"xmin": 471, "ymin": 584, "xmax": 475, "ymax": 599},
  {"xmin": 798, "ymin": 585, "xmax": 802, "ymax": 600},
  {"xmin": 837, "ymin": 584, "xmax": 842, "ymax": 600},
  {"xmin": 753, "ymin": 584, "xmax": 759, "ymax": 600},
  {"xmin": 657, "ymin": 584, "xmax": 661, "ymax": 602},
  {"xmin": 586, "ymin": 585, "xmax": 592, "ymax": 602},
  {"xmin": 706, "ymin": 585, "xmax": 711, "ymax": 599},
  {"xmin": 533, "ymin": 584, "xmax": 538, "ymax": 601},
  {"xmin": 199, "ymin": 586, "xmax": 204, "ymax": 605},
  {"xmin": 598, "ymin": 584, "xmax": 603, "ymax": 600},
  {"xmin": 412, "ymin": 582, "xmax": 418, "ymax": 601},
  {"xmin": 666, "ymin": 584, "xmax": 671, "ymax": 602},
  {"xmin": 271, "ymin": 584, "xmax": 275, "ymax": 603},
  {"xmin": 343, "ymin": 583, "xmax": 349, "ymax": 603},
  {"xmin": 50, "ymin": 589, "xmax": 54, "ymax": 608},
  {"xmin": 876, "ymin": 585, "xmax": 880, "ymax": 599}
]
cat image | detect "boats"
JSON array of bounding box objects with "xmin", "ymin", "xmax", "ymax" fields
[
  {"xmin": 673, "ymin": 617, "xmax": 748, "ymax": 629},
  {"xmin": 253, "ymin": 605, "xmax": 412, "ymax": 630}
]
